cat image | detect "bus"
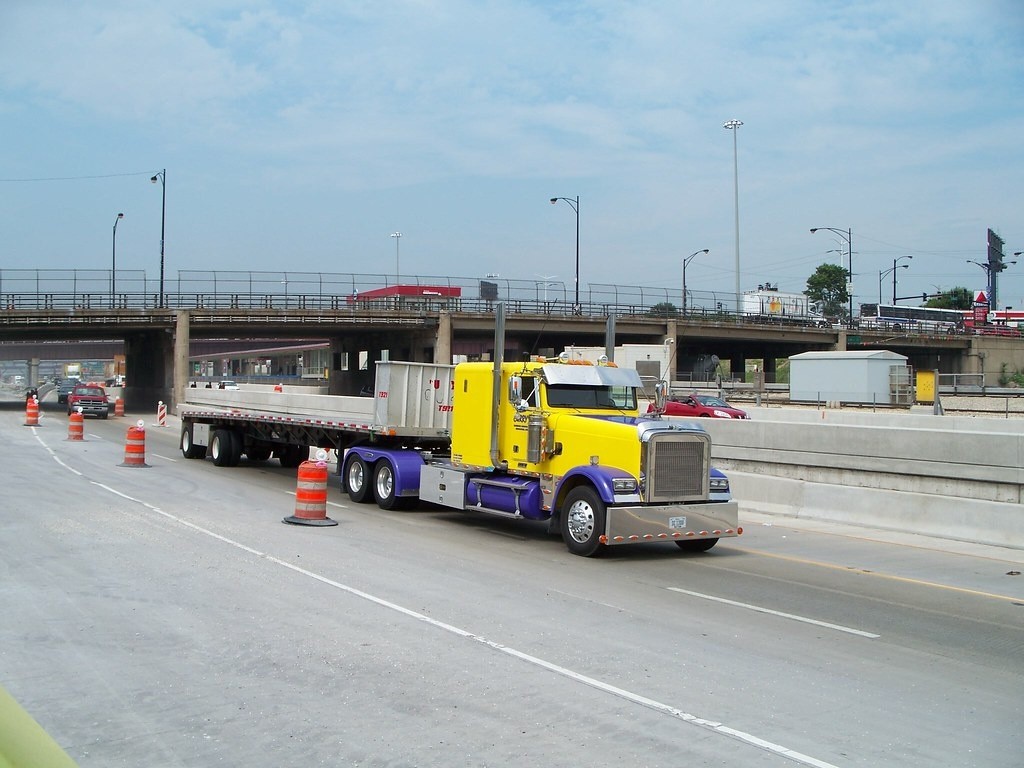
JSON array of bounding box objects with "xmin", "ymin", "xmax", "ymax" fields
[{"xmin": 859, "ymin": 304, "xmax": 965, "ymax": 335}]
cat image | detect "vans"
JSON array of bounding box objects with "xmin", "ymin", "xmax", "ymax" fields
[{"xmin": 116, "ymin": 376, "xmax": 125, "ymax": 386}]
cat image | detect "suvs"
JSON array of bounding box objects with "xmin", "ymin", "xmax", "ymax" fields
[{"xmin": 68, "ymin": 385, "xmax": 110, "ymax": 419}]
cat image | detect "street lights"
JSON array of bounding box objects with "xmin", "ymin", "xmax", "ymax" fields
[
  {"xmin": 534, "ymin": 273, "xmax": 557, "ymax": 307},
  {"xmin": 810, "ymin": 228, "xmax": 854, "ymax": 328},
  {"xmin": 993, "ymin": 261, "xmax": 1016, "ymax": 312},
  {"xmin": 150, "ymin": 169, "xmax": 166, "ymax": 308},
  {"xmin": 113, "ymin": 212, "xmax": 123, "ymax": 309},
  {"xmin": 894, "ymin": 256, "xmax": 911, "ymax": 305},
  {"xmin": 966, "ymin": 259, "xmax": 990, "ymax": 314},
  {"xmin": 1005, "ymin": 307, "xmax": 1013, "ymax": 326},
  {"xmin": 550, "ymin": 197, "xmax": 579, "ymax": 312},
  {"xmin": 880, "ymin": 265, "xmax": 909, "ymax": 303},
  {"xmin": 392, "ymin": 231, "xmax": 403, "ymax": 306},
  {"xmin": 682, "ymin": 249, "xmax": 709, "ymax": 317}
]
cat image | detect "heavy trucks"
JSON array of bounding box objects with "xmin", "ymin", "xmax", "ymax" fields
[
  {"xmin": 177, "ymin": 302, "xmax": 745, "ymax": 557},
  {"xmin": 743, "ymin": 291, "xmax": 829, "ymax": 329}
]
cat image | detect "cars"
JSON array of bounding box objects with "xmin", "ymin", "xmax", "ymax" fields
[
  {"xmin": 831, "ymin": 318, "xmax": 858, "ymax": 331},
  {"xmin": 647, "ymin": 394, "xmax": 751, "ymax": 421},
  {"xmin": 983, "ymin": 325, "xmax": 1022, "ymax": 337},
  {"xmin": 51, "ymin": 377, "xmax": 85, "ymax": 404},
  {"xmin": 104, "ymin": 377, "xmax": 116, "ymax": 387}
]
